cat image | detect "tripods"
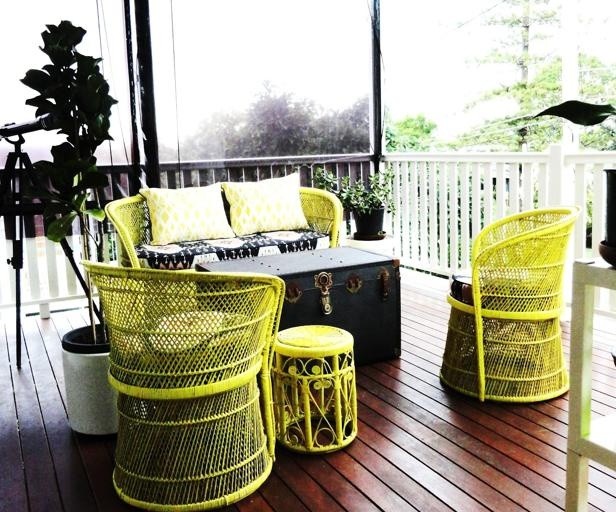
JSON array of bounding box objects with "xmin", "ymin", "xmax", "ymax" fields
[{"xmin": 0, "ymin": 144, "xmax": 104, "ymax": 371}]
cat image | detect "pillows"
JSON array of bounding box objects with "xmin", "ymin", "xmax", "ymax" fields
[{"xmin": 138, "ymin": 169, "xmax": 309, "ymax": 246}]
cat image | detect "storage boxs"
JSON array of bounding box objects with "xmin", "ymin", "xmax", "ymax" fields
[{"xmin": 197, "ymin": 245, "xmax": 403, "ymax": 364}]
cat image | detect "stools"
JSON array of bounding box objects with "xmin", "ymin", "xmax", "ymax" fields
[{"xmin": 274, "ymin": 325, "xmax": 357, "ymax": 456}]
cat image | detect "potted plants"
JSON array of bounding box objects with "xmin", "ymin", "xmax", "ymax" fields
[
  {"xmin": 314, "ymin": 168, "xmax": 399, "ymax": 241},
  {"xmin": 21, "ymin": 19, "xmax": 121, "ymax": 438}
]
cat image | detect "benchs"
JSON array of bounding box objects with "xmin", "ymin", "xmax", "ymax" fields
[{"xmin": 103, "ymin": 184, "xmax": 344, "ymax": 268}]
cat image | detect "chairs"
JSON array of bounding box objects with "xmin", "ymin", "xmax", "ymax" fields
[
  {"xmin": 438, "ymin": 206, "xmax": 578, "ymax": 403},
  {"xmin": 80, "ymin": 260, "xmax": 286, "ymax": 511}
]
